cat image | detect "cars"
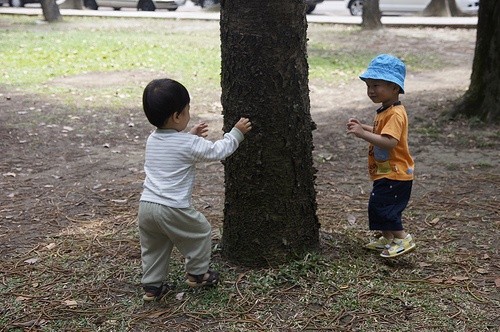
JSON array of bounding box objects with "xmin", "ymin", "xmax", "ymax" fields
[{"xmin": 345, "ymin": 0, "xmax": 479, "ymax": 16}]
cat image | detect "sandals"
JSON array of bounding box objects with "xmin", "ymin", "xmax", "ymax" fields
[
  {"xmin": 186, "ymin": 270, "xmax": 220, "ymax": 287},
  {"xmin": 380, "ymin": 234, "xmax": 416, "ymax": 257},
  {"xmin": 143, "ymin": 281, "xmax": 176, "ymax": 301},
  {"xmin": 363, "ymin": 236, "xmax": 393, "ymax": 251}
]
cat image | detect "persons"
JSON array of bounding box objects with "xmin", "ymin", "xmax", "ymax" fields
[
  {"xmin": 138, "ymin": 79, "xmax": 252, "ymax": 301},
  {"xmin": 345, "ymin": 54, "xmax": 416, "ymax": 257}
]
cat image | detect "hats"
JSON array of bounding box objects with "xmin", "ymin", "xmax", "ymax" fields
[{"xmin": 359, "ymin": 54, "xmax": 406, "ymax": 94}]
